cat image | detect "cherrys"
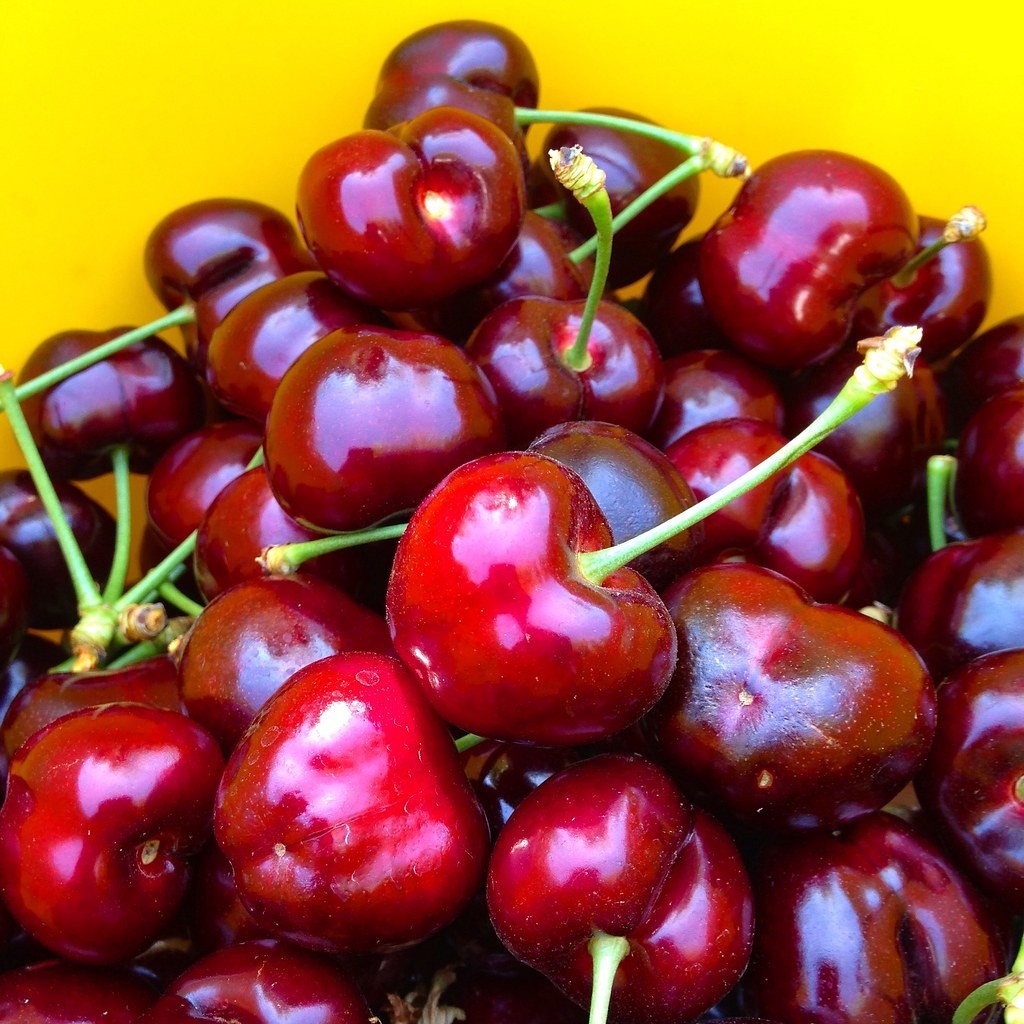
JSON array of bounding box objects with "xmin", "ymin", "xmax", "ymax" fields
[{"xmin": 0, "ymin": 18, "xmax": 1024, "ymax": 1024}]
